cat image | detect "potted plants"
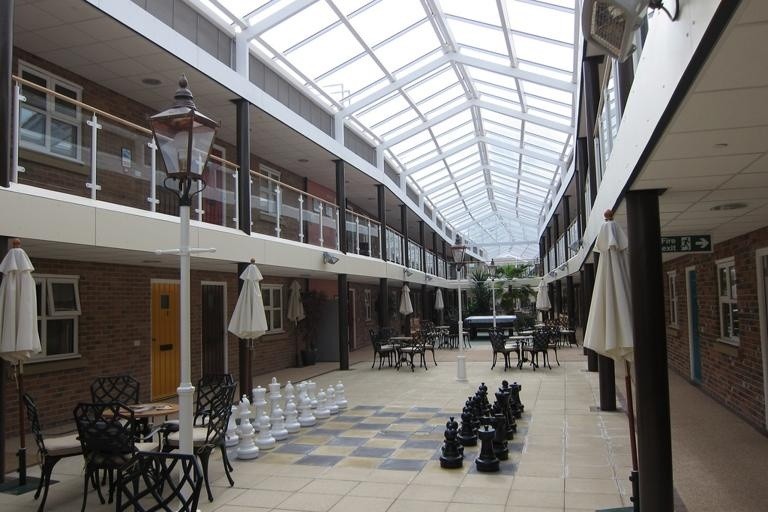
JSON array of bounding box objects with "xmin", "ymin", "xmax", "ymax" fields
[{"xmin": 297, "ymin": 288, "xmax": 331, "ymax": 367}]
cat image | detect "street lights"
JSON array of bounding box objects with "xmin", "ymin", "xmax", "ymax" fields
[
  {"xmin": 449, "ymin": 233, "xmax": 472, "ymax": 381},
  {"xmin": 144, "ymin": 69, "xmax": 222, "ymax": 512},
  {"xmin": 488, "ymin": 258, "xmax": 500, "ymax": 336}
]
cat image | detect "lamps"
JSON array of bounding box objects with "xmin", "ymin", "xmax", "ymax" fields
[
  {"xmin": 323, "ymin": 251, "xmax": 340, "ymax": 265},
  {"xmin": 549, "ymin": 269, "xmax": 558, "ymax": 277},
  {"xmin": 558, "ymin": 262, "xmax": 569, "ymax": 272},
  {"xmin": 582, "ymin": 0, "xmax": 679, "ymax": 64},
  {"xmin": 425, "ymin": 273, "xmax": 433, "ymax": 280},
  {"xmin": 567, "ymin": 240, "xmax": 584, "ymax": 252},
  {"xmin": 403, "ymin": 267, "xmax": 413, "ymax": 277}
]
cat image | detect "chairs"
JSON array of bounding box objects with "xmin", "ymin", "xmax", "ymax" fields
[
  {"xmin": 488, "ymin": 311, "xmax": 579, "ymax": 371},
  {"xmin": 368, "ymin": 316, "xmax": 471, "ymax": 373},
  {"xmin": 23, "ymin": 373, "xmax": 238, "ymax": 512}
]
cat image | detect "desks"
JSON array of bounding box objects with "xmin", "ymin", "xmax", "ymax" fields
[{"xmin": 464, "ymin": 315, "xmax": 519, "ymax": 340}]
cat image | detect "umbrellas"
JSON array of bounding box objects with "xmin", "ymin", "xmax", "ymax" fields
[
  {"xmin": 435, "ymin": 288, "xmax": 445, "ymax": 326},
  {"xmin": 287, "ymin": 278, "xmax": 306, "ymax": 367},
  {"xmin": 0, "ymin": 238, "xmax": 42, "ymax": 485},
  {"xmin": 229, "ymin": 258, "xmax": 268, "ymax": 398},
  {"xmin": 398, "ymin": 283, "xmax": 414, "ymax": 338},
  {"xmin": 536, "ymin": 278, "xmax": 552, "ymax": 322},
  {"xmin": 582, "ymin": 210, "xmax": 638, "ymax": 473}
]
